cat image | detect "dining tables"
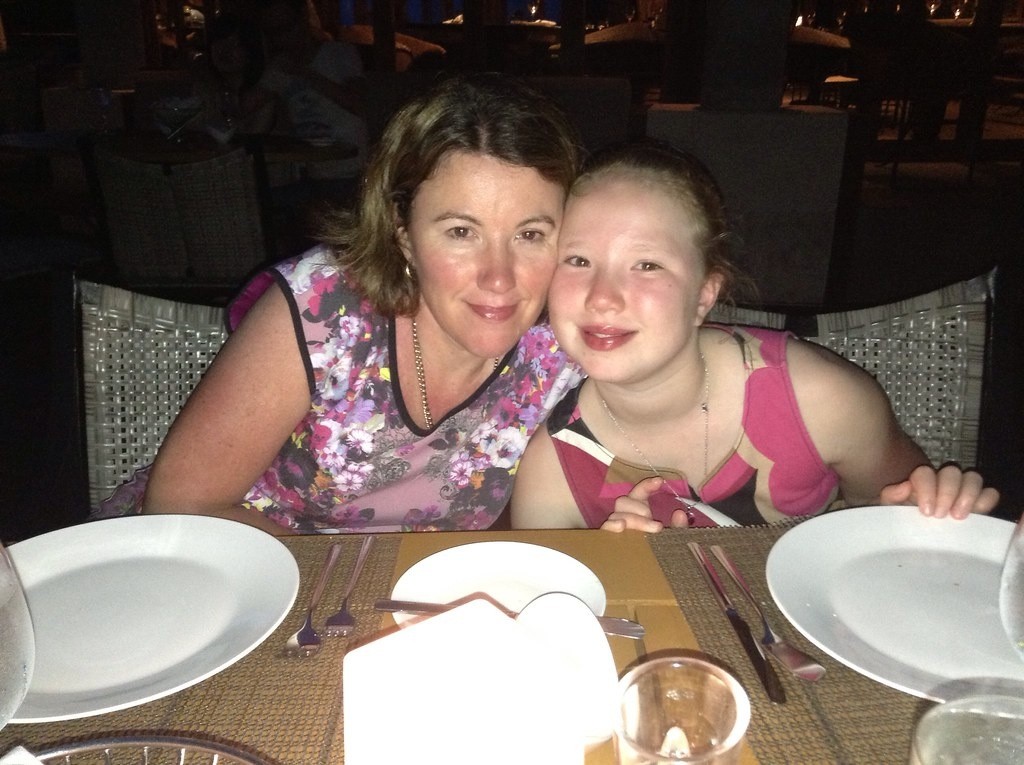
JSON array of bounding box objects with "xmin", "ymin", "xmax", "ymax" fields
[{"xmin": 0, "ymin": 524, "xmax": 938, "ymax": 765}]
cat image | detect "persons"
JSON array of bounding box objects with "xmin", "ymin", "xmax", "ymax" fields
[
  {"xmin": 89, "ymin": 66, "xmax": 589, "ymax": 536},
  {"xmin": 244, "ymin": 0, "xmax": 368, "ymax": 234},
  {"xmin": 176, "ymin": 11, "xmax": 277, "ymax": 134},
  {"xmin": 511, "ymin": 132, "xmax": 999, "ymax": 535}
]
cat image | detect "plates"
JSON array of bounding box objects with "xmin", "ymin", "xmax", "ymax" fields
[
  {"xmin": 0, "ymin": 514, "xmax": 299, "ymax": 724},
  {"xmin": 390, "ymin": 542, "xmax": 606, "ymax": 627},
  {"xmin": 763, "ymin": 504, "xmax": 1024, "ymax": 718}
]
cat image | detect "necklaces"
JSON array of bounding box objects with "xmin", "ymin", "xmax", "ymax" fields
[
  {"xmin": 593, "ymin": 344, "xmax": 710, "ymax": 527},
  {"xmin": 410, "ymin": 313, "xmax": 498, "ymax": 431}
]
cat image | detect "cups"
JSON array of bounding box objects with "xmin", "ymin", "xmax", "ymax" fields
[
  {"xmin": 905, "ymin": 694, "xmax": 1024, "ymax": 765},
  {"xmin": 614, "ymin": 658, "xmax": 752, "ymax": 765}
]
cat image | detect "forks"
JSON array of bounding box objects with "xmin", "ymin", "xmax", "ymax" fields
[
  {"xmin": 275, "ymin": 546, "xmax": 339, "ymax": 660},
  {"xmin": 313, "ymin": 536, "xmax": 374, "ymax": 638}
]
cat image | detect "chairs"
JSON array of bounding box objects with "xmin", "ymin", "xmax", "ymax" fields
[
  {"xmin": 68, "ymin": 273, "xmax": 228, "ymax": 511},
  {"xmin": 82, "ymin": 133, "xmax": 266, "ymax": 290},
  {"xmin": 703, "ymin": 265, "xmax": 1002, "ymax": 470}
]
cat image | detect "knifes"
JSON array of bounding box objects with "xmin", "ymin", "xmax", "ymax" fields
[
  {"xmin": 375, "ymin": 599, "xmax": 645, "ymax": 640},
  {"xmin": 687, "ymin": 541, "xmax": 788, "ymax": 704},
  {"xmin": 711, "ymin": 544, "xmax": 827, "ymax": 682}
]
